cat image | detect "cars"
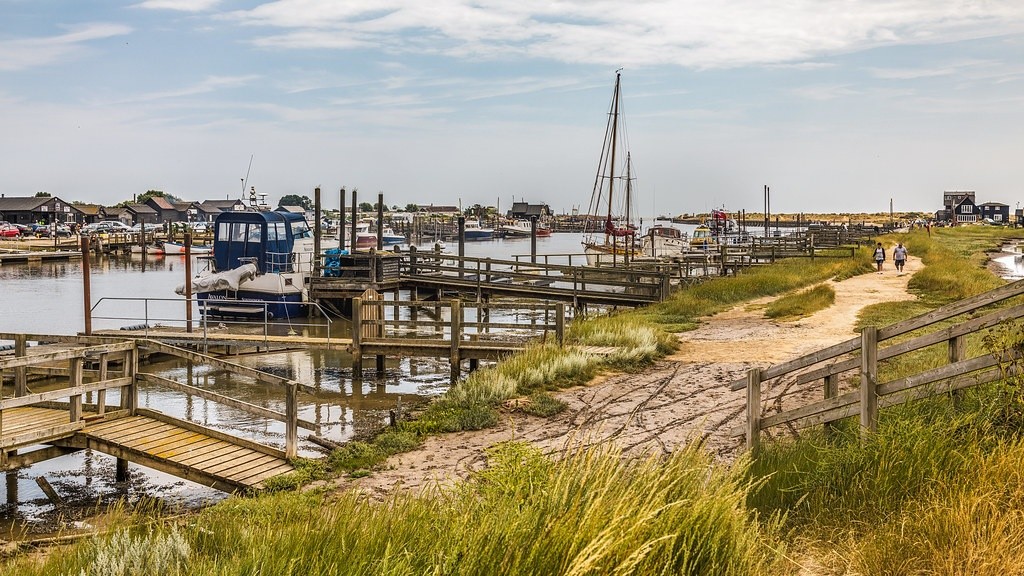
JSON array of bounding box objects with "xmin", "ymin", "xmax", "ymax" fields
[{"xmin": 0, "ymin": 219, "xmax": 216, "ymax": 239}]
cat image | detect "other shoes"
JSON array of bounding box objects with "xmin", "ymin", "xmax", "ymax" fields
[
  {"xmin": 877, "ymin": 272, "xmax": 879, "ymax": 274},
  {"xmin": 900, "ymin": 272, "xmax": 903, "ymax": 275},
  {"xmin": 881, "ymin": 272, "xmax": 883, "ymax": 274},
  {"xmin": 896, "ymin": 272, "xmax": 899, "ymax": 276}
]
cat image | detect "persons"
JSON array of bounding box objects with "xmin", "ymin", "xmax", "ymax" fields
[
  {"xmin": 893, "ymin": 243, "xmax": 907, "ymax": 272},
  {"xmin": 873, "ymin": 242, "xmax": 886, "ymax": 270},
  {"xmin": 908, "ymin": 219, "xmax": 945, "ymax": 228}
]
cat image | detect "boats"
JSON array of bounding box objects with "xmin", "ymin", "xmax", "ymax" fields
[
  {"xmin": 146, "ymin": 245, "xmax": 164, "ymax": 255},
  {"xmin": 502, "ymin": 218, "xmax": 532, "ymax": 239},
  {"xmin": 163, "ymin": 242, "xmax": 213, "ymax": 254},
  {"xmin": 580, "ymin": 64, "xmax": 749, "ymax": 268},
  {"xmin": 536, "ymin": 225, "xmax": 551, "ymax": 237},
  {"xmin": 464, "ymin": 220, "xmax": 494, "ymax": 241},
  {"xmin": 383, "ymin": 228, "xmax": 405, "ymax": 246},
  {"xmin": 355, "ymin": 231, "xmax": 378, "ymax": 252},
  {"xmin": 195, "ymin": 207, "xmax": 344, "ymax": 322}
]
